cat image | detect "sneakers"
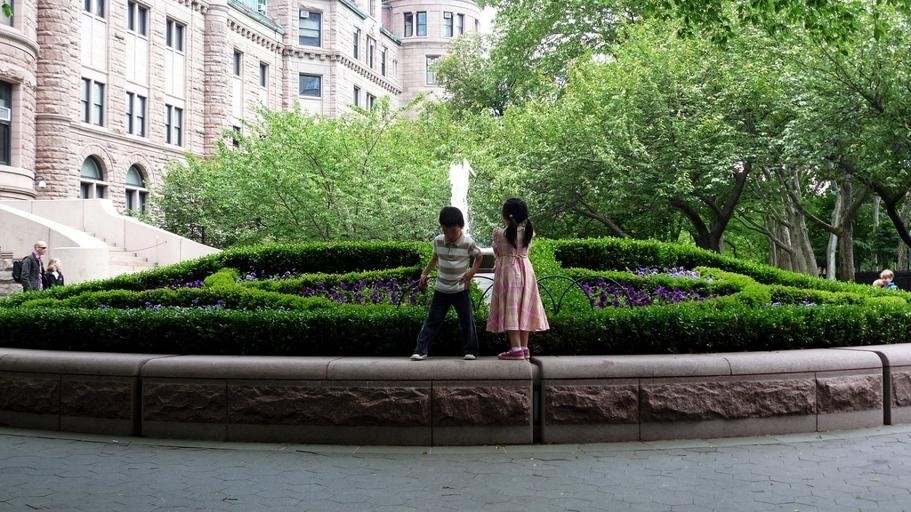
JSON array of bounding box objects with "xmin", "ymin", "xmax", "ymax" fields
[
  {"xmin": 411, "ymin": 353, "xmax": 427, "ymax": 360},
  {"xmin": 497, "ymin": 350, "xmax": 525, "ymax": 360},
  {"xmin": 464, "ymin": 355, "xmax": 476, "ymax": 359},
  {"xmin": 522, "ymin": 348, "xmax": 530, "ymax": 358}
]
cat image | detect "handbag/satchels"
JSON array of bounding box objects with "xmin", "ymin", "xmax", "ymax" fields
[{"xmin": 13, "ymin": 261, "xmax": 22, "ymax": 283}]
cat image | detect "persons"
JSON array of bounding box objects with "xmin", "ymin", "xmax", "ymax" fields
[
  {"xmin": 881, "ymin": 268, "xmax": 896, "ymax": 294},
  {"xmin": 20, "ymin": 240, "xmax": 42, "ymax": 289},
  {"xmin": 873, "ymin": 279, "xmax": 885, "ymax": 289},
  {"xmin": 48, "ymin": 257, "xmax": 66, "ymax": 286},
  {"xmin": 491, "ymin": 198, "xmax": 549, "ymax": 357},
  {"xmin": 408, "ymin": 206, "xmax": 483, "ymax": 362}
]
